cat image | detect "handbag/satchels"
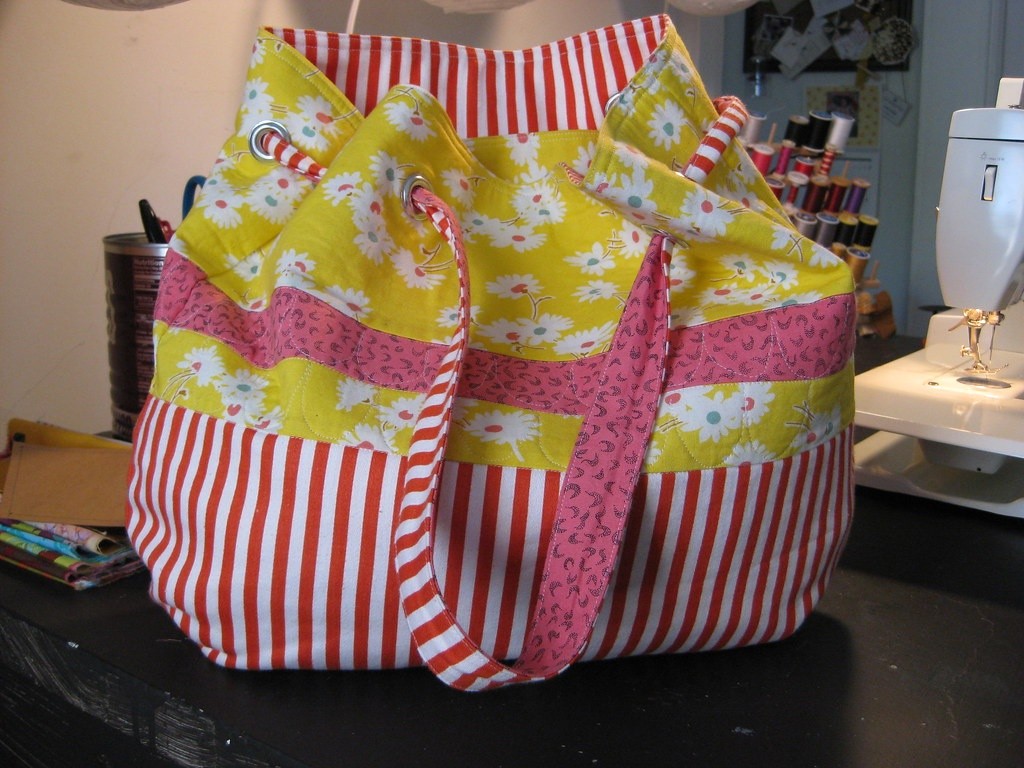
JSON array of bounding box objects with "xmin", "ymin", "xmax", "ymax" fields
[{"xmin": 124, "ymin": 12, "xmax": 859, "ymax": 695}]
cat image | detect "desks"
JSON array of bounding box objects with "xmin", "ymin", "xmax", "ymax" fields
[{"xmin": 1, "ymin": 331, "xmax": 1024, "ymax": 766}]
